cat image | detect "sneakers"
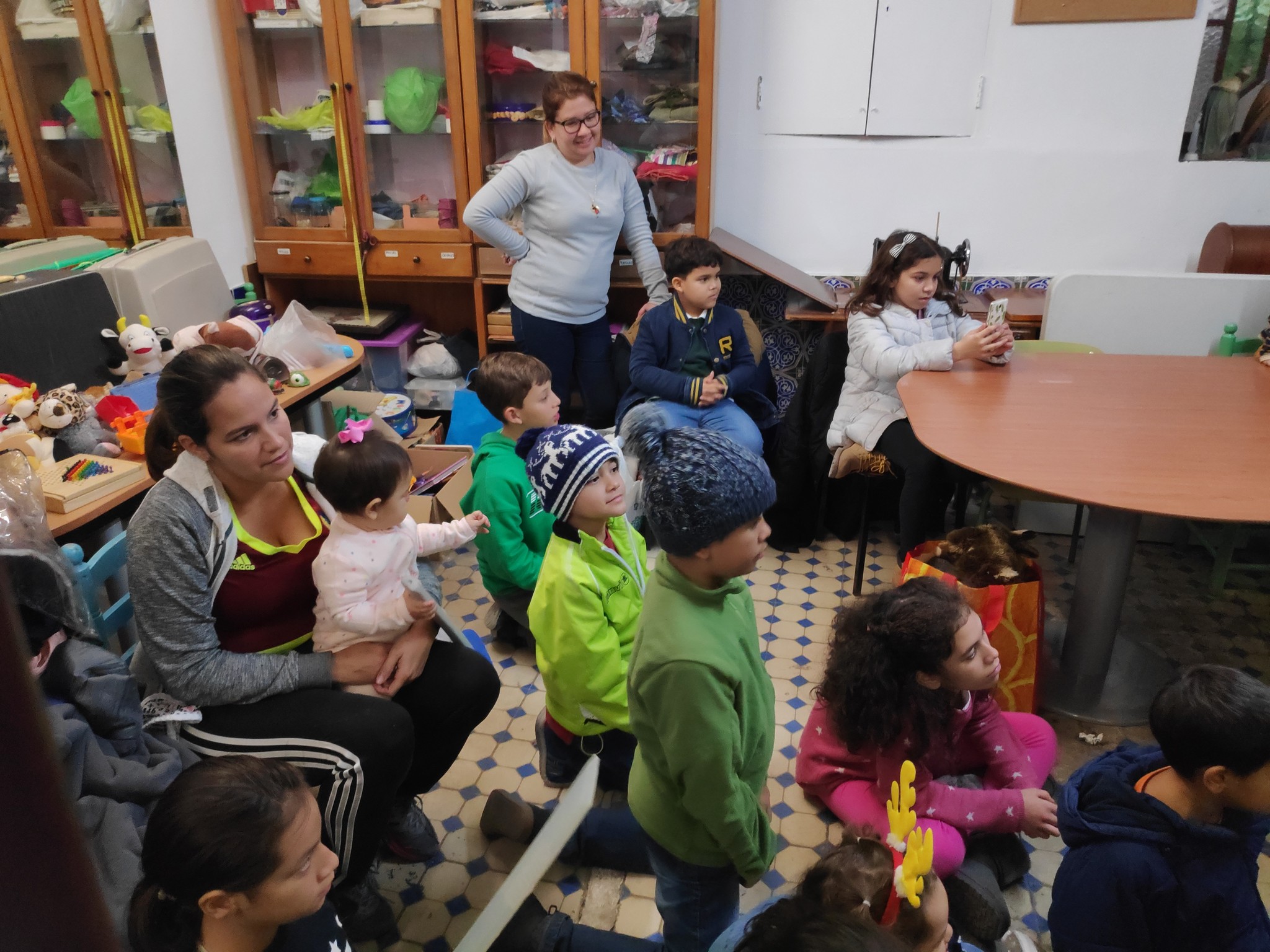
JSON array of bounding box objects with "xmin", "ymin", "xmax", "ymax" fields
[
  {"xmin": 336, "ymin": 872, "xmax": 397, "ymax": 942},
  {"xmin": 388, "ymin": 795, "xmax": 440, "ymax": 861}
]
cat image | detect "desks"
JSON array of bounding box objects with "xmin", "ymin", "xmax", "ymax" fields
[
  {"xmin": 897, "ymin": 353, "xmax": 1270, "ymax": 727},
  {"xmin": 784, "ymin": 286, "xmax": 1045, "ymax": 542},
  {"xmin": 46, "ymin": 334, "xmax": 364, "ymax": 538}
]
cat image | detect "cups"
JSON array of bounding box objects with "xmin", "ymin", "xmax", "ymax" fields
[
  {"xmin": 437, "ymin": 198, "xmax": 458, "ymax": 229},
  {"xmin": 367, "ymin": 99, "xmax": 386, "ymax": 120},
  {"xmin": 62, "ymin": 198, "xmax": 84, "ymax": 227}
]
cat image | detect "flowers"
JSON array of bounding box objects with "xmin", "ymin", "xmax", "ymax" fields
[{"xmin": 338, "ymin": 418, "xmax": 373, "ymax": 443}]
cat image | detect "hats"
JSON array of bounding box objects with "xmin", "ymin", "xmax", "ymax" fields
[
  {"xmin": 515, "ymin": 424, "xmax": 620, "ymax": 522},
  {"xmin": 618, "ymin": 402, "xmax": 777, "ymax": 556}
]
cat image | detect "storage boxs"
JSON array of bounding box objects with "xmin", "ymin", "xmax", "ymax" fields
[
  {"xmin": 255, "ymin": 10, "xmax": 304, "ymax": 20},
  {"xmin": 487, "ymin": 299, "xmax": 512, "ymax": 326},
  {"xmin": 341, "ymin": 315, "xmax": 426, "ymax": 393},
  {"xmin": 488, "ymin": 325, "xmax": 512, "ymax": 335},
  {"xmin": 253, "ymin": 19, "xmax": 313, "ymax": 29},
  {"xmin": 405, "ymin": 448, "xmax": 475, "ymax": 562},
  {"xmin": 360, "ymin": 8, "xmax": 438, "ymax": 26}
]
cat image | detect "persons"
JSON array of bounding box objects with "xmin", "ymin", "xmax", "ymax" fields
[
  {"xmin": 514, "ymin": 425, "xmax": 649, "ymax": 792},
  {"xmin": 461, "ymin": 73, "xmax": 672, "ymax": 431},
  {"xmin": 480, "ymin": 410, "xmax": 776, "ymax": 952},
  {"xmin": 708, "ymin": 757, "xmax": 983, "ymax": 952},
  {"xmin": 614, "ymin": 236, "xmax": 767, "ymax": 457},
  {"xmin": 794, "ymin": 573, "xmax": 1059, "ymax": 939},
  {"xmin": 311, "ymin": 419, "xmax": 490, "ymax": 701},
  {"xmin": 127, "ymin": 346, "xmax": 501, "ymax": 945},
  {"xmin": 827, "ymin": 229, "xmax": 1015, "ymax": 566},
  {"xmin": 1047, "ymin": 664, "xmax": 1270, "ymax": 952},
  {"xmin": 124, "ymin": 756, "xmax": 359, "ymax": 952},
  {"xmin": 460, "ymin": 351, "xmax": 560, "ymax": 646}
]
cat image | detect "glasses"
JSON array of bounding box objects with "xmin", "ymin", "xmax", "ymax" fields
[{"xmin": 549, "ymin": 107, "xmax": 601, "ymax": 134}]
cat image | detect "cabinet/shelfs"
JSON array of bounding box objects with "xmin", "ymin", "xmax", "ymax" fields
[
  {"xmin": 0, "ymin": 0, "xmax": 192, "ymax": 249},
  {"xmin": 214, "ymin": 0, "xmax": 713, "ymax": 360}
]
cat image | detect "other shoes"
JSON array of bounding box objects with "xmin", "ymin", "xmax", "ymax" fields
[
  {"xmin": 487, "ymin": 892, "xmax": 558, "ymax": 952},
  {"xmin": 536, "ymin": 706, "xmax": 573, "ymax": 789},
  {"xmin": 479, "ymin": 788, "xmax": 548, "ymax": 843}
]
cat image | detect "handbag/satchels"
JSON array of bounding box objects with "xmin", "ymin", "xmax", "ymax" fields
[
  {"xmin": 258, "ymin": 300, "xmax": 347, "ymax": 373},
  {"xmin": 406, "ymin": 329, "xmax": 462, "ymax": 380},
  {"xmin": 897, "ymin": 540, "xmax": 1044, "ymax": 714}
]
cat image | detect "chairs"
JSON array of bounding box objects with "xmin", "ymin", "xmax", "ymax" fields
[
  {"xmin": 60, "ymin": 530, "xmax": 138, "ymax": 665},
  {"xmin": 770, "ymin": 330, "xmax": 900, "ymax": 596}
]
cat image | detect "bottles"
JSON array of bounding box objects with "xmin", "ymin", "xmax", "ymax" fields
[
  {"xmin": 39, "ymin": 120, "xmax": 66, "ymax": 140},
  {"xmin": 268, "ymin": 190, "xmax": 294, "ymax": 227},
  {"xmin": 291, "ymin": 196, "xmax": 330, "ymax": 227}
]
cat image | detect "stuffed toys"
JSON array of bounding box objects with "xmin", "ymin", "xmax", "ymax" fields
[{"xmin": 0, "ymin": 313, "xmax": 261, "ymax": 471}]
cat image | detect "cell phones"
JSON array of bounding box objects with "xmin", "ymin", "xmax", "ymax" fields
[{"xmin": 985, "ymin": 297, "xmax": 1008, "ymax": 328}]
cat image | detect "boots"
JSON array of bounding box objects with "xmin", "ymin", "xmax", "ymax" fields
[
  {"xmin": 828, "ymin": 441, "xmax": 897, "ymax": 478},
  {"xmin": 946, "ymin": 831, "xmax": 1030, "ymax": 944}
]
